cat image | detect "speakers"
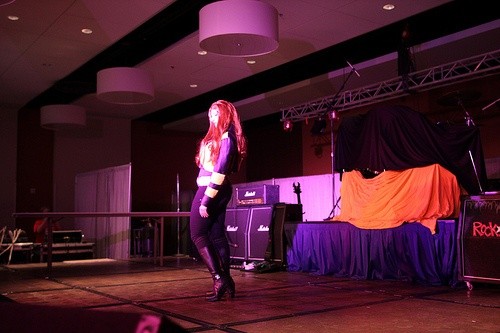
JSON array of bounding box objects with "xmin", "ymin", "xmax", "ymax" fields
[
  {"xmin": 457, "ymin": 193, "xmax": 500, "ymax": 284},
  {"xmin": 225, "ymin": 203, "xmax": 303, "ymax": 266}
]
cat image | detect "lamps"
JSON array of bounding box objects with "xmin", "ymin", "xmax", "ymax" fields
[
  {"xmin": 96, "ymin": 67, "xmax": 153, "ymax": 105},
  {"xmin": 40, "ymin": 105, "xmax": 88, "ymax": 131},
  {"xmin": 199, "ymin": 0, "xmax": 278, "ymax": 57}
]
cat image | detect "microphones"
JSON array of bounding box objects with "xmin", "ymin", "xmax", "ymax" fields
[
  {"xmin": 346, "ymin": 60, "xmax": 361, "ymax": 77},
  {"xmin": 466, "ymin": 111, "xmax": 476, "ymax": 126}
]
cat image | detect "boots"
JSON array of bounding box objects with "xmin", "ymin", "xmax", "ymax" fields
[{"xmin": 198, "ymin": 244, "xmax": 236, "ymax": 302}]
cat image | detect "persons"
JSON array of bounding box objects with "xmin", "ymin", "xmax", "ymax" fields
[
  {"xmin": 33, "ymin": 206, "xmax": 48, "ymax": 243},
  {"xmin": 190, "ymin": 100, "xmax": 246, "ymax": 300}
]
